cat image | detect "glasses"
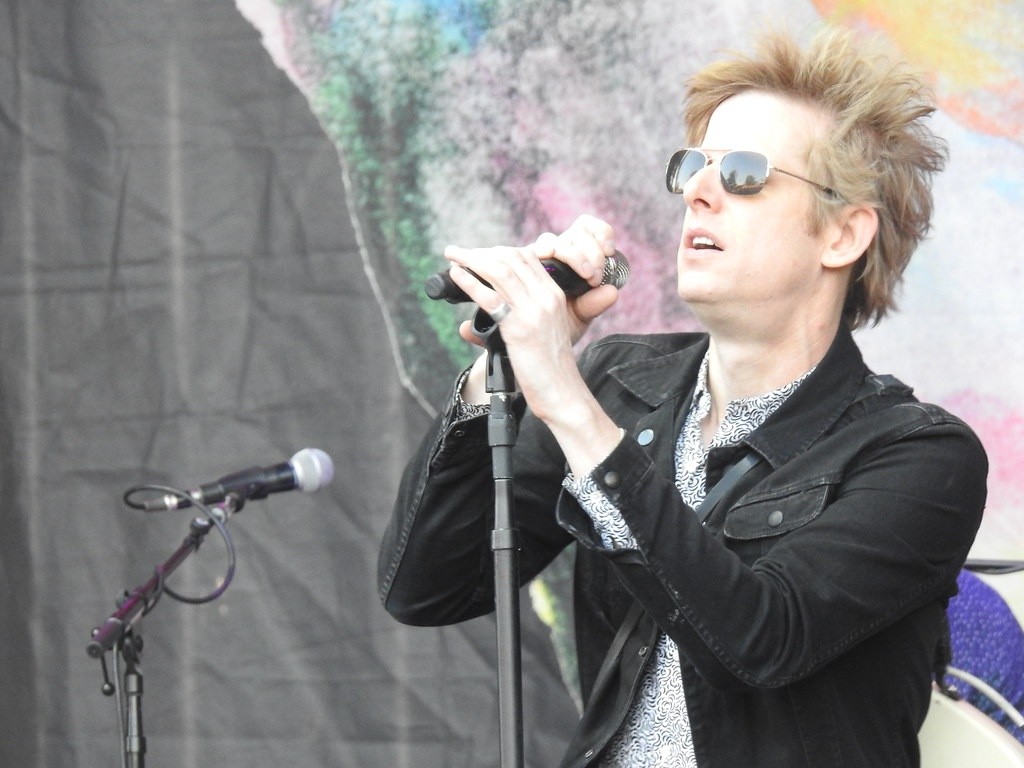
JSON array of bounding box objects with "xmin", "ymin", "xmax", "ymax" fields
[{"xmin": 665, "ymin": 148, "xmax": 851, "ymax": 204}]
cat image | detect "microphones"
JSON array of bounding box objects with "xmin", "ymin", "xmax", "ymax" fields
[
  {"xmin": 143, "ymin": 447, "xmax": 335, "ymax": 511},
  {"xmin": 424, "ymin": 250, "xmax": 630, "ymax": 305}
]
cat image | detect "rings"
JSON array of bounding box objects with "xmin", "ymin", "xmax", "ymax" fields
[{"xmin": 490, "ymin": 304, "xmax": 511, "ymax": 324}]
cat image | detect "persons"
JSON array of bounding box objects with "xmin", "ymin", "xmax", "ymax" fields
[{"xmin": 375, "ymin": 37, "xmax": 989, "ymax": 768}]
